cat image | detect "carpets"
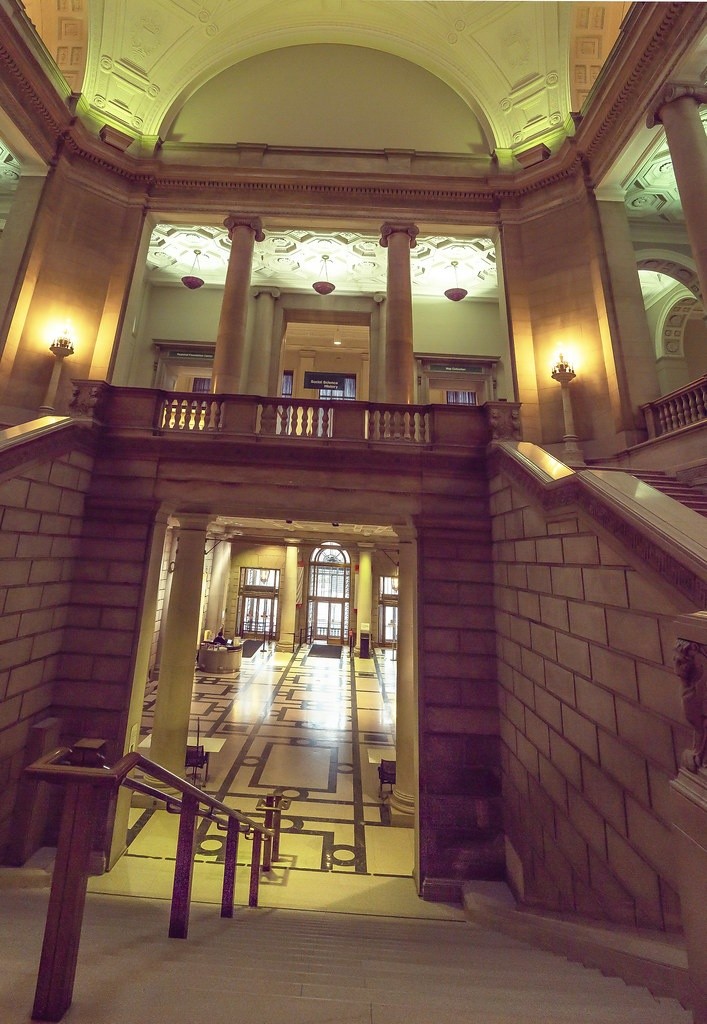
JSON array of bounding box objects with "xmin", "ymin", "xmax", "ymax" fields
[
  {"xmin": 307, "ymin": 644, "xmax": 342, "ymax": 659},
  {"xmin": 242, "ymin": 640, "xmax": 264, "ymax": 657}
]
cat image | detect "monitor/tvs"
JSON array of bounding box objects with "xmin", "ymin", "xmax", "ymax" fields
[{"xmin": 227, "ymin": 639, "xmax": 232, "ymax": 644}]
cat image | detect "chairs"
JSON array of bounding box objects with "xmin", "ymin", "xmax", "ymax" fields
[
  {"xmin": 378, "ymin": 759, "xmax": 396, "ymax": 799},
  {"xmin": 185, "ymin": 745, "xmax": 210, "ymax": 781}
]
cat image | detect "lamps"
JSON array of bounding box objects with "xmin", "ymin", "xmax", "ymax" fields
[
  {"xmin": 545, "ymin": 339, "xmax": 585, "ymax": 466},
  {"xmin": 181, "ymin": 250, "xmax": 204, "ymax": 289},
  {"xmin": 444, "ymin": 261, "xmax": 468, "ymax": 301},
  {"xmin": 37, "ymin": 316, "xmax": 78, "ymax": 418},
  {"xmin": 313, "ymin": 255, "xmax": 336, "ymax": 295}
]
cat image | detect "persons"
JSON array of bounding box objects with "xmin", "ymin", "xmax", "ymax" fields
[{"xmin": 213, "ymin": 632, "xmax": 226, "ymax": 645}]
